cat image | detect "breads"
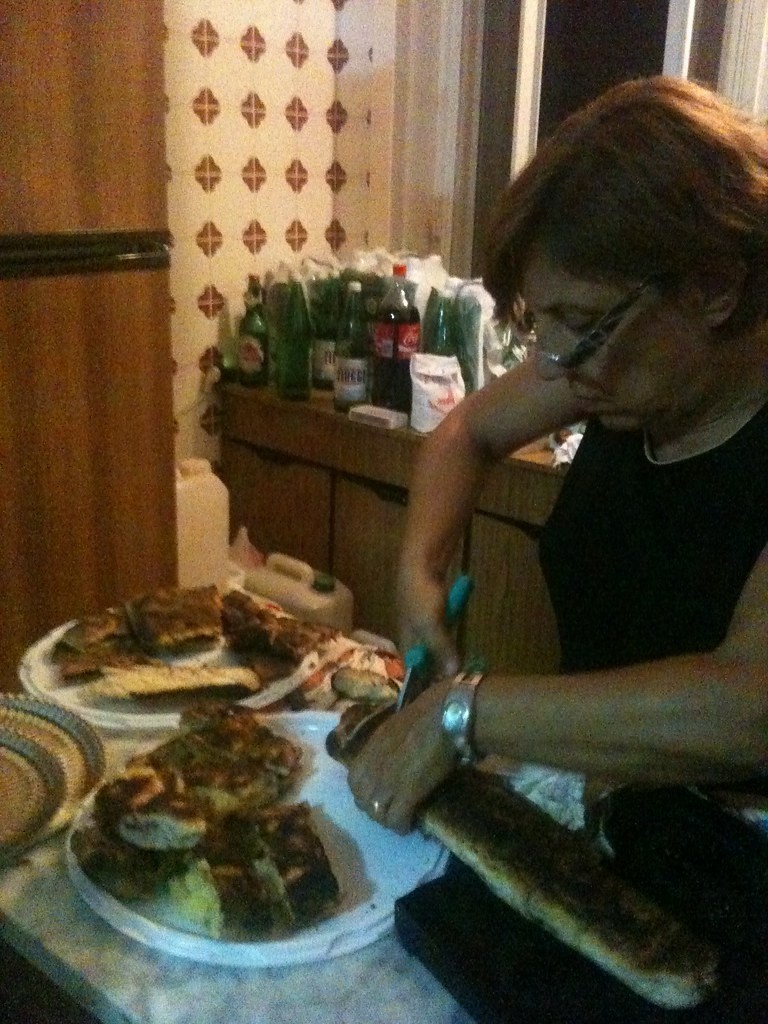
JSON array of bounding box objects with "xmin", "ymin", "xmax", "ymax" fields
[
  {"xmin": 70, "ymin": 704, "xmax": 342, "ymax": 938},
  {"xmin": 328, "ymin": 701, "xmax": 726, "ymax": 1008},
  {"xmin": 57, "ymin": 583, "xmax": 340, "ymax": 701}
]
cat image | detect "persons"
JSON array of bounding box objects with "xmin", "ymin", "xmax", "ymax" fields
[{"xmin": 345, "ymin": 74, "xmax": 767, "ymax": 966}]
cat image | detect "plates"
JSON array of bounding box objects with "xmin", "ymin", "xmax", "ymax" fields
[
  {"xmin": 19, "ymin": 606, "xmax": 182, "ymax": 728},
  {"xmin": 0, "ymin": 693, "xmax": 103, "ymax": 863},
  {"xmin": 71, "ymin": 709, "xmax": 451, "ymax": 967}
]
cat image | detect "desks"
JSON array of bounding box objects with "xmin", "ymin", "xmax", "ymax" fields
[
  {"xmin": 0, "ymin": 691, "xmax": 583, "ymax": 1024},
  {"xmin": 213, "ymin": 381, "xmax": 570, "ymax": 679}
]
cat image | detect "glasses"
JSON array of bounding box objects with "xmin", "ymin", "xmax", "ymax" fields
[{"xmin": 509, "ymin": 256, "xmax": 674, "ymax": 371}]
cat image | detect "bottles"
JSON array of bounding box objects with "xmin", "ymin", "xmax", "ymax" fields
[{"xmin": 238, "ymin": 262, "xmax": 512, "ymax": 432}]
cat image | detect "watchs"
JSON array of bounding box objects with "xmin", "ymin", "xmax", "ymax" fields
[{"xmin": 440, "ymin": 671, "xmax": 484, "ymax": 772}]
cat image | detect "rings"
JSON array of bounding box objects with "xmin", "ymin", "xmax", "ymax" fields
[{"xmin": 368, "ymin": 800, "xmax": 389, "ymax": 813}]
